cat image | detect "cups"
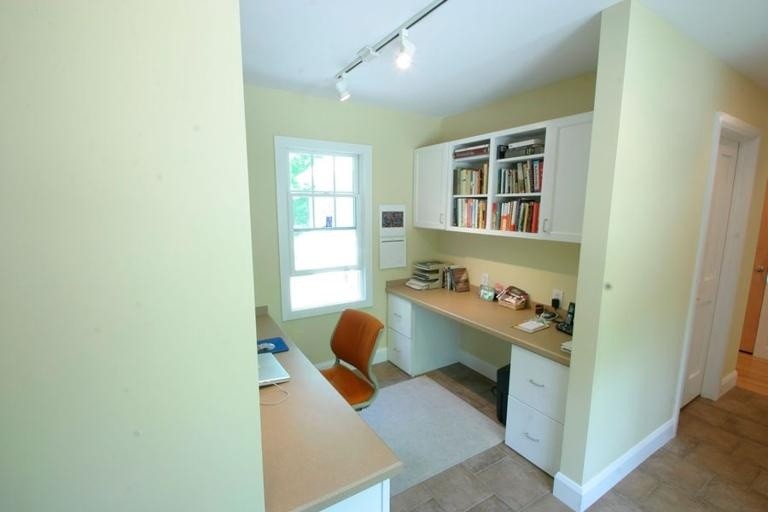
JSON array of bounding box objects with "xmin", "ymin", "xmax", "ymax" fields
[{"xmin": 326, "ymin": 216, "xmax": 333, "ymax": 228}]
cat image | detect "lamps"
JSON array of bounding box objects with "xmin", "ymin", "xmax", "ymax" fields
[{"xmin": 335, "ymin": 29, "xmax": 420, "ymax": 103}]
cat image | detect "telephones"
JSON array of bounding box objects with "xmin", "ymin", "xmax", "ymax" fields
[{"xmin": 556, "ymin": 302, "xmax": 575, "ymax": 336}]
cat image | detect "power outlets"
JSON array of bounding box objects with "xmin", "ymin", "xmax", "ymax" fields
[{"xmin": 551, "ymin": 288, "xmax": 563, "ymax": 308}]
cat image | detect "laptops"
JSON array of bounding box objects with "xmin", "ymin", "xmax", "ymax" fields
[{"xmin": 258, "ymin": 352, "xmax": 290, "ymax": 386}]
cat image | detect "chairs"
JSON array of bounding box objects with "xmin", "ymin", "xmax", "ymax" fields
[{"xmin": 316, "ymin": 307, "xmax": 386, "ymax": 412}]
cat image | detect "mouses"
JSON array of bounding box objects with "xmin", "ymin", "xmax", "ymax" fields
[{"xmin": 257, "ymin": 343, "xmax": 274, "ymax": 353}]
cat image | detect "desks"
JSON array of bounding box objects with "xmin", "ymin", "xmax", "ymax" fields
[
  {"xmin": 255, "ymin": 313, "xmax": 402, "ymax": 511},
  {"xmin": 387, "ymin": 279, "xmax": 575, "ymax": 479}
]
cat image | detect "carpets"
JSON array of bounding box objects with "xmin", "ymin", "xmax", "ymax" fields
[{"xmin": 356, "ymin": 374, "xmax": 505, "ymax": 500}]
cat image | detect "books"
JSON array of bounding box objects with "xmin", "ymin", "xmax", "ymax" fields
[
  {"xmin": 500, "ymin": 160, "xmax": 542, "ymax": 193},
  {"xmin": 492, "ymin": 199, "xmax": 540, "ymax": 233},
  {"xmin": 453, "ymin": 161, "xmax": 488, "ymax": 194},
  {"xmin": 405, "ymin": 261, "xmax": 469, "ymax": 292},
  {"xmin": 456, "ymin": 198, "xmax": 487, "ymax": 228}
]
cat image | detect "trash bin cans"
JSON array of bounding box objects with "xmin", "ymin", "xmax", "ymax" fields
[{"xmin": 496, "ymin": 364, "xmax": 510, "ymax": 428}]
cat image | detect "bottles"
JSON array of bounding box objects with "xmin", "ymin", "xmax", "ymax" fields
[{"xmin": 444, "ymin": 267, "xmax": 452, "ymax": 290}]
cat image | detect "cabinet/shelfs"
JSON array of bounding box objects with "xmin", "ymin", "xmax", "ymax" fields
[
  {"xmin": 539, "ymin": 111, "xmax": 594, "ymax": 244},
  {"xmin": 413, "ymin": 143, "xmax": 446, "ymax": 229},
  {"xmin": 491, "ymin": 120, "xmax": 550, "ymax": 237},
  {"xmin": 446, "ymin": 132, "xmax": 492, "ymax": 235}
]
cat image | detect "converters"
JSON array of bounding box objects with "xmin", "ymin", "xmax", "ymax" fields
[{"xmin": 552, "ymin": 299, "xmax": 559, "ymax": 308}]
